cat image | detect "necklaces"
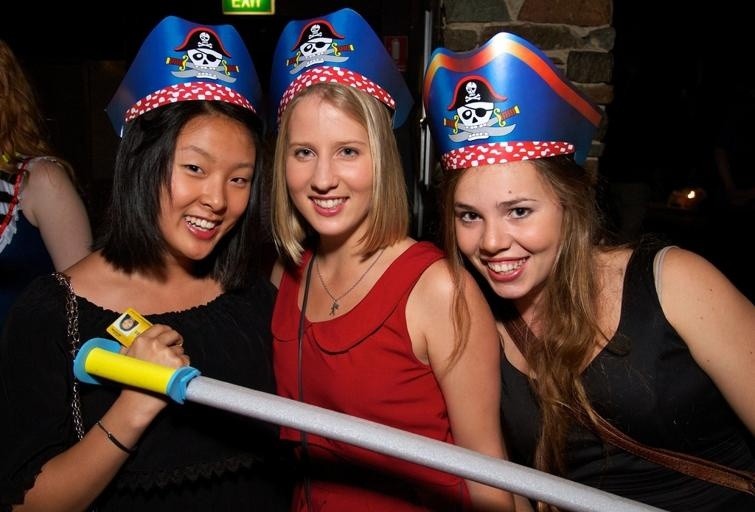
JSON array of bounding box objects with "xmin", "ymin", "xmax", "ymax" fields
[
  {"xmin": 1, "ymin": 150, "xmax": 22, "ymax": 165},
  {"xmin": 312, "ymin": 238, "xmax": 390, "ymax": 318}
]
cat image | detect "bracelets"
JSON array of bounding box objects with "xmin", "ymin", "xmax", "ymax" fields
[{"xmin": 96, "ymin": 418, "xmax": 134, "ymax": 456}]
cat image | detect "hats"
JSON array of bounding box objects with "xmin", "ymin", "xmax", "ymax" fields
[
  {"xmin": 412, "ymin": 30, "xmax": 607, "ymax": 172},
  {"xmin": 103, "ymin": 14, "xmax": 270, "ymax": 140},
  {"xmin": 265, "ymin": 7, "xmax": 415, "ymax": 131}
]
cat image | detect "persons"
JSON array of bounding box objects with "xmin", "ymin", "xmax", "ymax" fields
[
  {"xmin": 423, "ymin": 29, "xmax": 755, "ymax": 510},
  {"xmin": 0, "ymin": 14, "xmax": 271, "ymax": 512},
  {"xmin": 264, "ymin": 7, "xmax": 516, "ymax": 512},
  {"xmin": 2, "ymin": 44, "xmax": 95, "ymax": 316},
  {"xmin": 118, "ymin": 313, "xmax": 138, "ymax": 333}
]
